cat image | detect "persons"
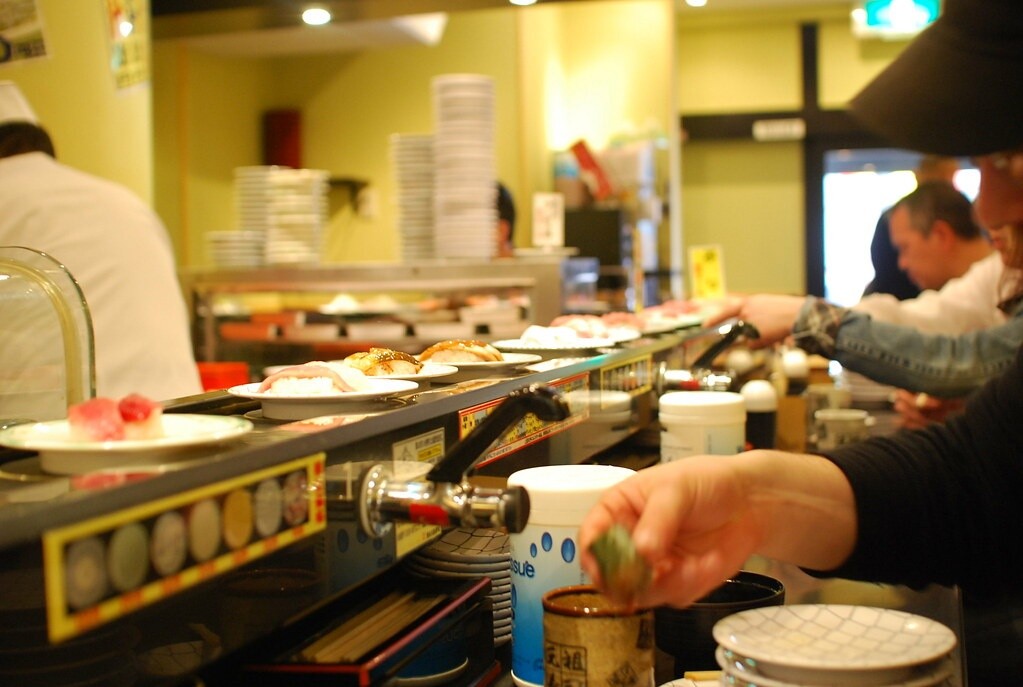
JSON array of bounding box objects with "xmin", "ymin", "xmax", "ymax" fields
[
  {"xmin": 497, "ymin": 183, "xmax": 515, "ymax": 257},
  {"xmin": 580, "ymin": 177, "xmax": 1023, "ymax": 687},
  {"xmin": 0, "ymin": 81, "xmax": 206, "ymax": 425}
]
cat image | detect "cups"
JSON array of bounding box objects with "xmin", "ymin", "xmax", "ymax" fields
[{"xmin": 813, "ymin": 407, "xmax": 873, "ymax": 447}]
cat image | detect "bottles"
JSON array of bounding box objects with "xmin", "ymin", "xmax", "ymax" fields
[
  {"xmin": 506, "ymin": 464, "xmax": 647, "ymax": 687},
  {"xmin": 658, "ymin": 388, "xmax": 746, "ymax": 465}
]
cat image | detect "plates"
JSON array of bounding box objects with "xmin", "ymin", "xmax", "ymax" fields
[
  {"xmin": 226, "ymin": 375, "xmax": 420, "ymax": 418},
  {"xmin": 390, "ymin": 366, "xmax": 459, "ymax": 392},
  {"xmin": 429, "ymin": 353, "xmax": 544, "ymax": 384},
  {"xmin": 401, "ymin": 472, "xmax": 514, "ymax": 649},
  {"xmin": 0, "ymin": 413, "xmax": 253, "ymax": 476},
  {"xmin": 614, "ymin": 300, "xmax": 713, "ymax": 343},
  {"xmin": 713, "ymin": 603, "xmax": 955, "ymax": 687},
  {"xmin": 845, "ymin": 366, "xmax": 893, "ymax": 411},
  {"xmin": 207, "ymin": 163, "xmax": 334, "ymax": 272},
  {"xmin": 491, "ymin": 338, "xmax": 620, "ymax": 363},
  {"xmin": 386, "ymin": 72, "xmax": 503, "ymax": 259}
]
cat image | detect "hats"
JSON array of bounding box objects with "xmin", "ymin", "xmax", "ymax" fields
[
  {"xmin": 0, "ymin": 80, "xmax": 38, "ymax": 128},
  {"xmin": 848, "ymin": 0, "xmax": 1023, "ymax": 155}
]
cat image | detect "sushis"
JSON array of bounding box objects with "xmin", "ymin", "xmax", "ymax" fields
[
  {"xmin": 256, "ymin": 301, "xmax": 695, "ymax": 396},
  {"xmin": 67, "ymin": 393, "xmax": 167, "ymax": 444}
]
